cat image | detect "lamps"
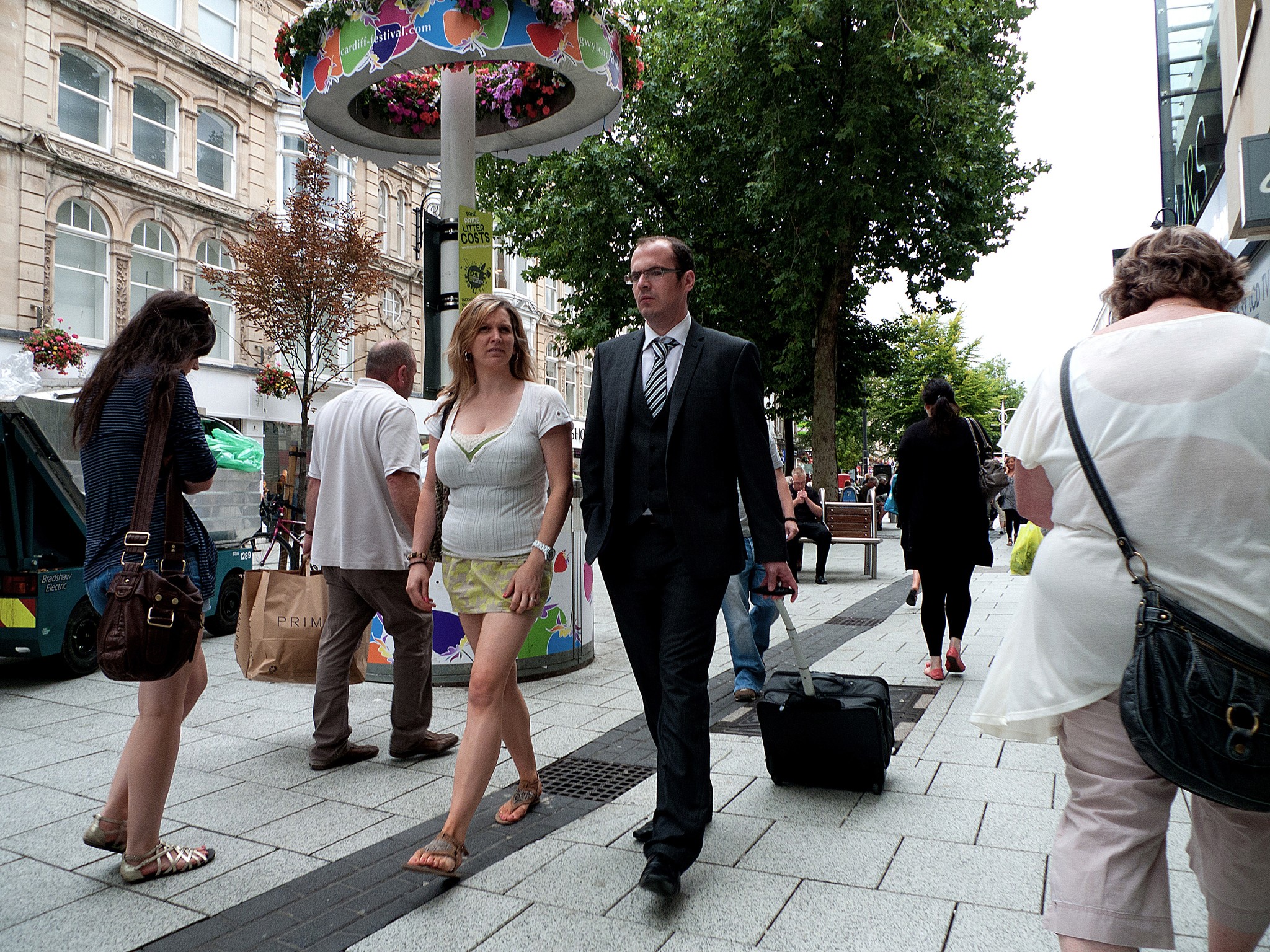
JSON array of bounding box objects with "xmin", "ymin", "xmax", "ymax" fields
[{"xmin": 1150, "ymin": 207, "xmax": 1177, "ymax": 229}]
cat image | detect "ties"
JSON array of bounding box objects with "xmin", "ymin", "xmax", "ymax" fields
[{"xmin": 644, "ymin": 339, "xmax": 680, "ymax": 418}]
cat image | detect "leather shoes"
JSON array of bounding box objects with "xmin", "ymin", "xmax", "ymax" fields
[
  {"xmin": 633, "ymin": 819, "xmax": 654, "ymax": 842},
  {"xmin": 638, "ymin": 851, "xmax": 681, "ymax": 898},
  {"xmin": 815, "ymin": 575, "xmax": 827, "ymax": 585}
]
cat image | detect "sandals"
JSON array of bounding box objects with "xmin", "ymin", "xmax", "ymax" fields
[
  {"xmin": 83, "ymin": 814, "xmax": 127, "ymax": 853},
  {"xmin": 404, "ymin": 832, "xmax": 469, "ymax": 877},
  {"xmin": 120, "ymin": 839, "xmax": 216, "ymax": 885},
  {"xmin": 495, "ymin": 772, "xmax": 540, "ymax": 825}
]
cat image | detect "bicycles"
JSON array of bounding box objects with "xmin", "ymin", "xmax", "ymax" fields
[{"xmin": 239, "ymin": 491, "xmax": 322, "ymax": 572}]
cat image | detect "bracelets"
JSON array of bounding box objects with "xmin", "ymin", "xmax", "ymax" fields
[
  {"xmin": 407, "ymin": 552, "xmax": 427, "ymax": 561},
  {"xmin": 408, "ymin": 561, "xmax": 427, "ymax": 568},
  {"xmin": 785, "ymin": 517, "xmax": 797, "ymax": 523},
  {"xmin": 305, "ymin": 530, "xmax": 313, "ymax": 535}
]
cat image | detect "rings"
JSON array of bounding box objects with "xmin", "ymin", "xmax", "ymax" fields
[{"xmin": 529, "ymin": 598, "xmax": 536, "ymax": 602}]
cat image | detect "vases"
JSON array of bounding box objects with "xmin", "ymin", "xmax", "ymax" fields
[{"xmin": 299, "ymin": 1, "xmax": 620, "ymax": 153}]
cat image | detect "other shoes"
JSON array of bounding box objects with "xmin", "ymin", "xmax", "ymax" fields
[
  {"xmin": 989, "ymin": 526, "xmax": 1017, "ymax": 546},
  {"xmin": 876, "ymin": 522, "xmax": 882, "ymax": 531},
  {"xmin": 924, "ymin": 663, "xmax": 944, "ymax": 680},
  {"xmin": 945, "ymin": 647, "xmax": 966, "ymax": 673}
]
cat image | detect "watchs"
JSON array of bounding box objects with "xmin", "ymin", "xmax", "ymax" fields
[{"xmin": 532, "ymin": 539, "xmax": 556, "ymax": 562}]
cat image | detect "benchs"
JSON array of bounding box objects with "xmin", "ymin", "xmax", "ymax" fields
[{"xmin": 799, "ymin": 486, "xmax": 880, "ymax": 578}]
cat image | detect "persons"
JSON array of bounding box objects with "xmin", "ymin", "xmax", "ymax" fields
[
  {"xmin": 580, "ymin": 235, "xmax": 798, "ymax": 897},
  {"xmin": 844, "ymin": 473, "xmax": 891, "ymax": 505},
  {"xmin": 857, "ymin": 476, "xmax": 887, "ymax": 531},
  {"xmin": 277, "ymin": 470, "xmax": 287, "ymax": 495},
  {"xmin": 722, "ymin": 432, "xmax": 798, "ymax": 702},
  {"xmin": 65, "ymin": 291, "xmax": 218, "ymax": 884},
  {"xmin": 261, "ymin": 479, "xmax": 270, "ymax": 501},
  {"xmin": 990, "ymin": 456, "xmax": 1021, "ymax": 546},
  {"xmin": 301, "ymin": 338, "xmax": 459, "ymax": 772},
  {"xmin": 971, "ymin": 224, "xmax": 1269, "ymax": 952},
  {"xmin": 406, "ymin": 294, "xmax": 574, "ymax": 878},
  {"xmin": 787, "ymin": 467, "xmax": 832, "ymax": 584},
  {"xmin": 893, "ymin": 378, "xmax": 994, "ymax": 681}
]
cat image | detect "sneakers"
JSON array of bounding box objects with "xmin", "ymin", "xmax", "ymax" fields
[
  {"xmin": 389, "ymin": 729, "xmax": 458, "ymax": 758},
  {"xmin": 734, "ymin": 688, "xmax": 758, "ymax": 702},
  {"xmin": 310, "ymin": 743, "xmax": 379, "ymax": 771}
]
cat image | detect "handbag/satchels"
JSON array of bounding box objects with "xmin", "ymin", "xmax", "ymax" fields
[
  {"xmin": 965, "ymin": 416, "xmax": 1009, "ymax": 504},
  {"xmin": 427, "ymin": 390, "xmax": 460, "ymax": 563},
  {"xmin": 232, "ymin": 555, "xmax": 372, "ymax": 685},
  {"xmin": 1009, "ymin": 520, "xmax": 1044, "ymax": 576},
  {"xmin": 1119, "ymin": 589, "xmax": 1269, "ymax": 813},
  {"xmin": 883, "ymin": 473, "xmax": 899, "ymax": 515},
  {"xmin": 97, "ymin": 562, "xmax": 204, "ymax": 681},
  {"xmin": 996, "ymin": 495, "xmax": 1004, "ymax": 508}
]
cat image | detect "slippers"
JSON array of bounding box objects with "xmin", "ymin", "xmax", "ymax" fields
[{"xmin": 906, "ymin": 589, "xmax": 917, "ymax": 606}]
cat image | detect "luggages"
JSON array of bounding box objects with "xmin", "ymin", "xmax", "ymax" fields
[{"xmin": 746, "ymin": 585, "xmax": 895, "ymax": 796}]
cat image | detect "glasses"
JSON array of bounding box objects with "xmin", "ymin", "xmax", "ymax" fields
[
  {"xmin": 792, "ymin": 478, "xmax": 806, "ymax": 485},
  {"xmin": 622, "ymin": 267, "xmax": 685, "ymax": 285}
]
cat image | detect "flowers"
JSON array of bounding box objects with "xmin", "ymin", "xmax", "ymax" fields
[
  {"xmin": 18, "ymin": 318, "xmax": 89, "ymax": 378},
  {"xmin": 253, "ymin": 363, "xmax": 296, "ymax": 409},
  {"xmin": 274, "ymin": 1, "xmax": 644, "ymax": 133}
]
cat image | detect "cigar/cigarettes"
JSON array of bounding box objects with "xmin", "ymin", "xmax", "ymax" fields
[{"xmin": 800, "ymin": 488, "xmax": 802, "ymax": 491}]
